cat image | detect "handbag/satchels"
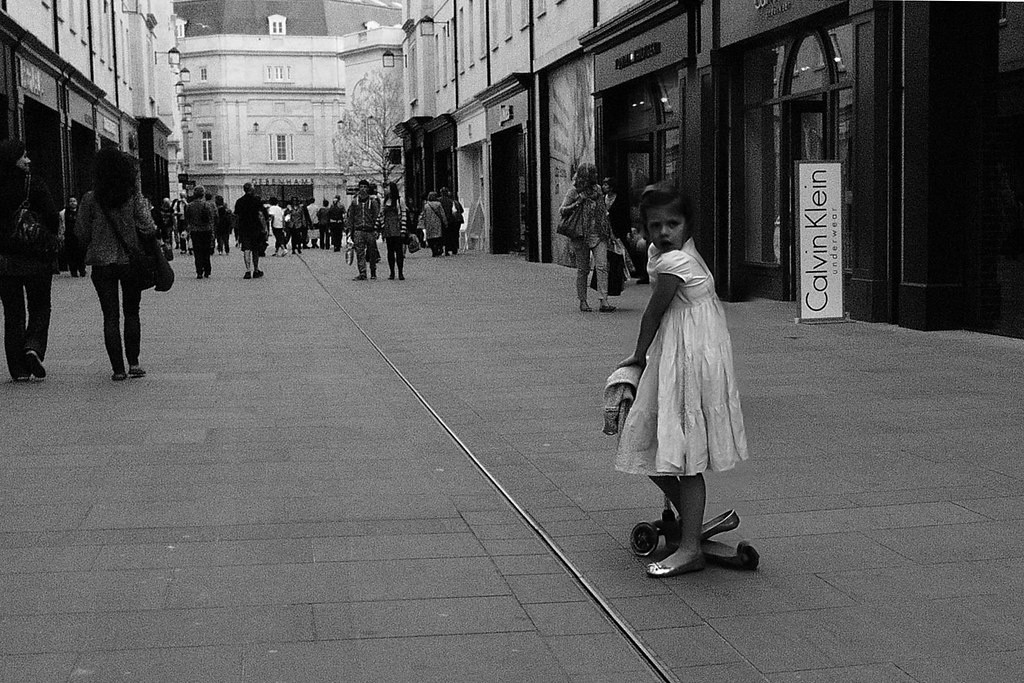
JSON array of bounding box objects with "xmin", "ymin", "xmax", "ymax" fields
[
  {"xmin": 400, "ymin": 221, "xmax": 410, "ymax": 246},
  {"xmin": 556, "ymin": 184, "xmax": 584, "ymax": 238},
  {"xmin": 441, "ymin": 221, "xmax": 449, "ymax": 235},
  {"xmin": 9, "ymin": 173, "xmax": 45, "ymax": 249},
  {"xmin": 417, "ymin": 219, "xmax": 426, "ymax": 230},
  {"xmin": 590, "ymin": 238, "xmax": 637, "ymax": 296},
  {"xmin": 130, "ymin": 254, "xmax": 155, "ymax": 291},
  {"xmin": 451, "ymin": 202, "xmax": 458, "ymax": 220},
  {"xmin": 307, "ymin": 228, "xmax": 320, "ymax": 240},
  {"xmin": 345, "ymin": 236, "xmax": 354, "ymax": 265}
]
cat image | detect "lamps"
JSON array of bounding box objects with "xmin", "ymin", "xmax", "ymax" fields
[
  {"xmin": 155, "ymin": 46, "xmax": 181, "ymax": 65},
  {"xmin": 171, "ymin": 67, "xmax": 192, "ymax": 83},
  {"xmin": 302, "ymin": 122, "xmax": 308, "ymax": 133},
  {"xmin": 337, "ymin": 119, "xmax": 344, "ymax": 129},
  {"xmin": 253, "ymin": 122, "xmax": 259, "ymax": 132},
  {"xmin": 419, "ymin": 14, "xmax": 448, "ymax": 36},
  {"xmin": 381, "ymin": 48, "xmax": 407, "ymax": 69}
]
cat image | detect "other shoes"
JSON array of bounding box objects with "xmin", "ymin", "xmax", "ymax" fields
[
  {"xmin": 579, "ymin": 301, "xmax": 592, "ymax": 311},
  {"xmin": 399, "ymin": 273, "xmax": 404, "ymax": 280},
  {"xmin": 445, "ymin": 252, "xmax": 450, "ymax": 257},
  {"xmin": 646, "ymin": 547, "xmax": 706, "ymax": 579},
  {"xmin": 129, "ymin": 367, "xmax": 145, "ymax": 375},
  {"xmin": 113, "ymin": 371, "xmax": 129, "ymax": 380},
  {"xmin": 371, "ymin": 273, "xmax": 377, "ymax": 279},
  {"xmin": 181, "ymin": 245, "xmax": 340, "ymax": 279},
  {"xmin": 353, "ymin": 275, "xmax": 367, "ymax": 281},
  {"xmin": 599, "ymin": 303, "xmax": 616, "ymax": 312},
  {"xmin": 26, "ymin": 350, "xmax": 48, "ymax": 378},
  {"xmin": 16, "ymin": 371, "xmax": 29, "ymax": 384},
  {"xmin": 390, "ymin": 272, "xmax": 394, "ymax": 279},
  {"xmin": 700, "ymin": 507, "xmax": 739, "ymax": 542}
]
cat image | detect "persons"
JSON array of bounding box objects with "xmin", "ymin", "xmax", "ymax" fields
[
  {"xmin": 74, "ymin": 148, "xmax": 158, "ymax": 381},
  {"xmin": 205, "ymin": 192, "xmax": 234, "ymax": 255},
  {"xmin": 58, "ymin": 197, "xmax": 87, "ymax": 277},
  {"xmin": 345, "ymin": 180, "xmax": 380, "ymax": 280},
  {"xmin": 0, "ymin": 140, "xmax": 65, "ymax": 379},
  {"xmin": 617, "ymin": 181, "xmax": 742, "ymax": 579},
  {"xmin": 266, "ymin": 195, "xmax": 347, "ymax": 257},
  {"xmin": 159, "ymin": 185, "xmax": 215, "ymax": 280},
  {"xmin": 234, "ymin": 182, "xmax": 270, "ymax": 279},
  {"xmin": 417, "ymin": 186, "xmax": 464, "ymax": 257},
  {"xmin": 380, "ymin": 182, "xmax": 411, "ymax": 281},
  {"xmin": 557, "ymin": 160, "xmax": 636, "ymax": 312}
]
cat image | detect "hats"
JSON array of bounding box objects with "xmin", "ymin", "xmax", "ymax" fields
[{"xmin": 0, "ymin": 141, "xmax": 25, "ymax": 167}]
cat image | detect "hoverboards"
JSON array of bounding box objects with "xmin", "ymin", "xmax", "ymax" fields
[{"xmin": 630, "ymin": 494, "xmax": 760, "ymax": 571}]
